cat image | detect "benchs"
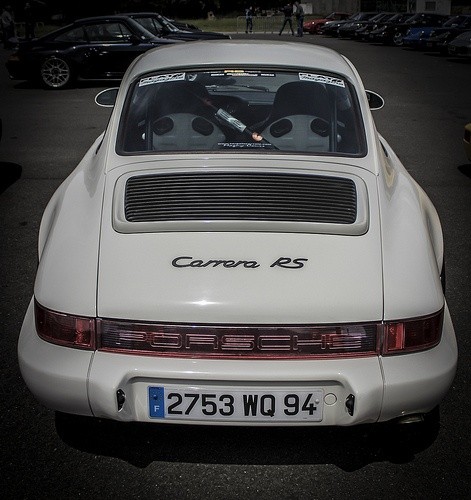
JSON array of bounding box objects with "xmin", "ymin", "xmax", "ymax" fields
[{"xmin": 142, "ymin": 113, "xmax": 342, "ymax": 152}]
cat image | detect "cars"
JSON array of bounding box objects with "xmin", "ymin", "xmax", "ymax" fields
[
  {"xmin": 447, "ymin": 31, "xmax": 471, "ymax": 61},
  {"xmin": 317, "ymin": 11, "xmax": 471, "ymax": 55},
  {"xmin": 16, "ymin": 39, "xmax": 459, "ymax": 452},
  {"xmin": 302, "ymin": 12, "xmax": 352, "ymax": 35},
  {"xmin": 5, "ymin": 15, "xmax": 187, "ymax": 90},
  {"xmin": 113, "ymin": 12, "xmax": 233, "ymax": 43}
]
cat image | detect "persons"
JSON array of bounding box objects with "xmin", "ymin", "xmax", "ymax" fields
[
  {"xmin": 278, "ymin": 1, "xmax": 295, "ymax": 36},
  {"xmin": 293, "ymin": 1, "xmax": 304, "ymax": 37},
  {"xmin": 1, "ymin": 8, "xmax": 14, "ymax": 49},
  {"xmin": 245, "ymin": 6, "xmax": 254, "ymax": 34}
]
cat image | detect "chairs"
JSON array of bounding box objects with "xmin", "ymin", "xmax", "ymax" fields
[
  {"xmin": 82, "ymin": 28, "xmax": 96, "ymax": 41},
  {"xmin": 102, "ymin": 24, "xmax": 121, "ymax": 40},
  {"xmin": 156, "ymin": 82, "xmax": 218, "ymax": 128},
  {"xmin": 270, "ymin": 81, "xmax": 334, "ymax": 124}
]
